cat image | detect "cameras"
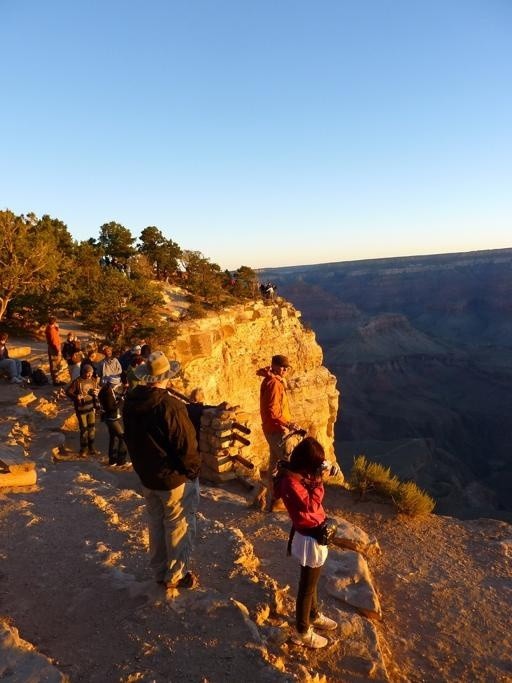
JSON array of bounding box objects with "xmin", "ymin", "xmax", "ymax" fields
[
  {"xmin": 319, "ymin": 459, "xmax": 339, "ymax": 478},
  {"xmin": 295, "ymin": 428, "xmax": 307, "ymax": 437}
]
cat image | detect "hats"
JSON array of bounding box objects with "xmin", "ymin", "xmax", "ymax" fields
[
  {"xmin": 81, "ymin": 364, "xmax": 94, "ymax": 373},
  {"xmin": 135, "ymin": 350, "xmax": 182, "ymax": 383},
  {"xmin": 272, "ymin": 355, "xmax": 292, "ymax": 368}
]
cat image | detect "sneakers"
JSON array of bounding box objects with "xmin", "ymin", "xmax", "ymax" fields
[
  {"xmin": 290, "ymin": 611, "xmax": 339, "ymax": 649},
  {"xmin": 80, "ymin": 450, "xmax": 101, "ymax": 458},
  {"xmin": 109, "ymin": 462, "xmax": 132, "ymax": 469}
]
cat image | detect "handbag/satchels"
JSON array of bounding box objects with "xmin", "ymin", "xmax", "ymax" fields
[
  {"xmin": 314, "ymin": 516, "xmax": 340, "ymax": 546},
  {"xmin": 32, "ymin": 368, "xmax": 47, "ymax": 385}
]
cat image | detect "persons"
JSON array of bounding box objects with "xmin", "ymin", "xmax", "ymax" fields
[
  {"xmin": 257, "ymin": 279, "xmax": 276, "ymax": 303},
  {"xmin": 258, "ymin": 355, "xmax": 301, "ymax": 502},
  {"xmin": 121, "ymin": 349, "xmax": 201, "ymax": 591},
  {"xmin": 279, "ymin": 436, "xmax": 338, "ymax": 649},
  {"xmin": 0, "ymin": 316, "xmax": 151, "ymax": 470}
]
